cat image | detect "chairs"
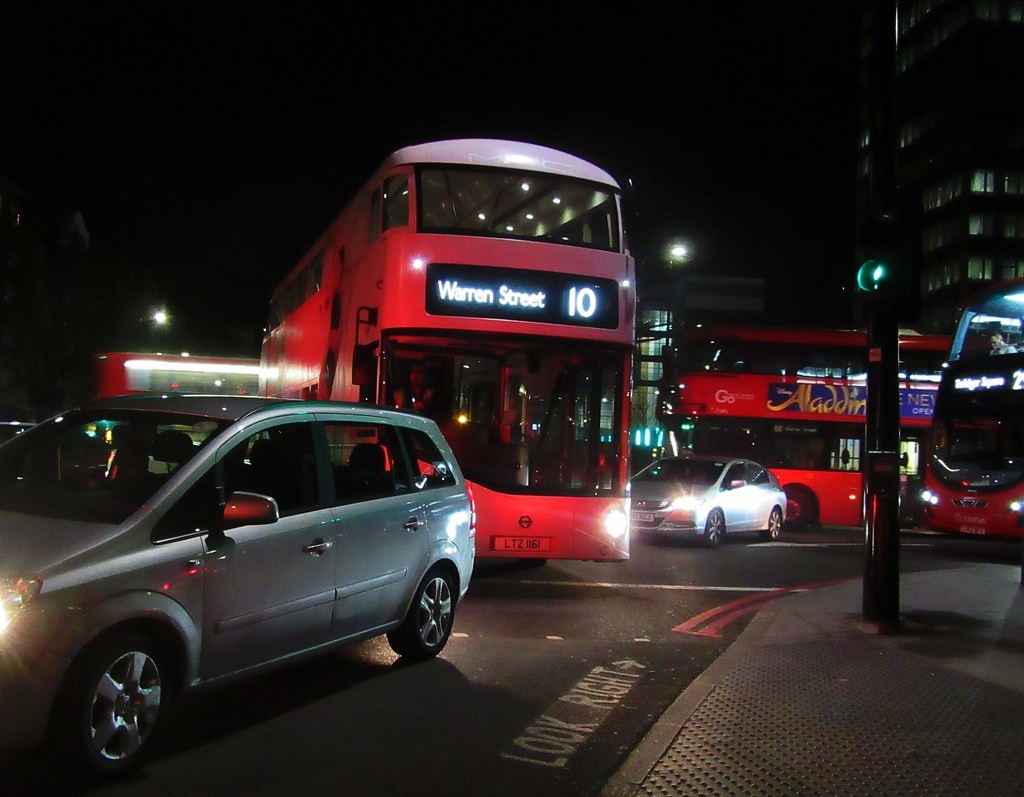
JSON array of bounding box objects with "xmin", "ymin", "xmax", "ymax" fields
[{"xmin": 133, "ymin": 429, "xmax": 196, "ymax": 503}]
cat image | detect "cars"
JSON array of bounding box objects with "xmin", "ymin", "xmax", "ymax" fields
[
  {"xmin": 630, "ymin": 454, "xmax": 789, "ymax": 548},
  {"xmin": 1, "ymin": 388, "xmax": 480, "ymax": 782}
]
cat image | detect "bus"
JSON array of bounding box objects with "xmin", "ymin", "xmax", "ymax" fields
[
  {"xmin": 83, "ymin": 350, "xmax": 262, "ymax": 494},
  {"xmin": 899, "ymin": 272, "xmax": 1023, "ymax": 543},
  {"xmin": 254, "ymin": 136, "xmax": 638, "ymax": 580},
  {"xmin": 637, "ymin": 321, "xmax": 1002, "ymax": 535}
]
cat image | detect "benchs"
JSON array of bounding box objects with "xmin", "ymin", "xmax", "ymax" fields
[{"xmin": 247, "ymin": 437, "xmax": 399, "ymax": 516}]
